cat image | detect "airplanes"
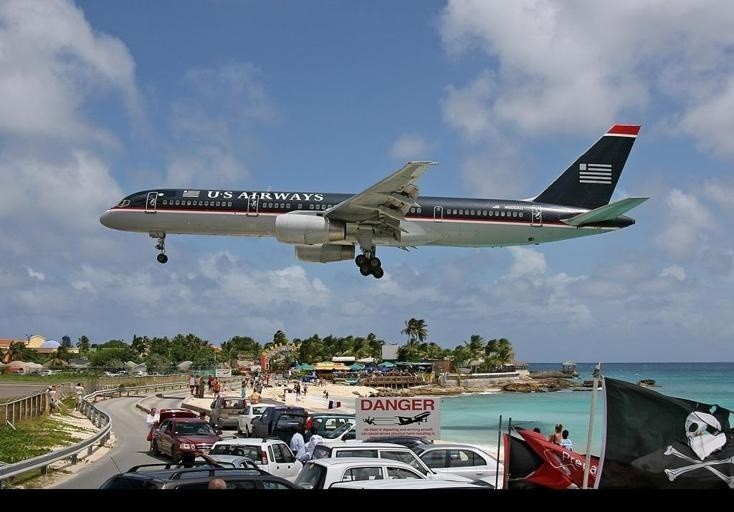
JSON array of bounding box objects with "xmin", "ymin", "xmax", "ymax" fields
[
  {"xmin": 396, "ymin": 412, "xmax": 430, "ymax": 427},
  {"xmin": 97, "ymin": 124, "xmax": 651, "ymax": 277}
]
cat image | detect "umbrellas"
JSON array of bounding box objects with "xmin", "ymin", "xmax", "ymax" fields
[{"xmin": 297, "ymin": 360, "xmax": 399, "ymax": 373}]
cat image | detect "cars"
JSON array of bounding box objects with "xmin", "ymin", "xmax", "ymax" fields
[
  {"xmin": 332, "ymin": 478, "xmax": 494, "ymax": 488},
  {"xmin": 209, "ymin": 397, "xmax": 252, "ymax": 431},
  {"xmin": 28, "ymin": 369, "xmax": 52, "ymax": 376},
  {"xmin": 174, "ymin": 455, "xmax": 260, "ymax": 470},
  {"xmin": 294, "ymin": 458, "xmax": 428, "ymax": 488},
  {"xmin": 238, "ymin": 403, "xmax": 278, "ymax": 438},
  {"xmin": 101, "ymin": 370, "xmax": 143, "ymax": 377},
  {"xmin": 407, "ymin": 443, "xmax": 505, "ymax": 479},
  {"xmin": 148, "ymin": 408, "xmax": 223, "ymax": 461},
  {"xmin": 317, "ymin": 422, "xmax": 434, "ymax": 449}
]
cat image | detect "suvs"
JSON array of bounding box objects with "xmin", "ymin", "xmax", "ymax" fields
[
  {"xmin": 310, "ymin": 440, "xmax": 473, "ymax": 483},
  {"xmin": 304, "ymin": 413, "xmax": 359, "ymax": 443},
  {"xmin": 251, "ymin": 405, "xmax": 309, "ymax": 441},
  {"xmin": 209, "ymin": 437, "xmax": 304, "ymax": 483},
  {"xmin": 100, "ymin": 461, "xmax": 305, "ymax": 488}
]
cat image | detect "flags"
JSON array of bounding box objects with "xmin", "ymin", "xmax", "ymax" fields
[
  {"xmin": 500, "ymin": 422, "xmax": 600, "ymax": 490},
  {"xmin": 593, "ymin": 370, "xmax": 733, "ymax": 490}
]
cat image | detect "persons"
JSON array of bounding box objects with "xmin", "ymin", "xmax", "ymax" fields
[
  {"xmin": 288, "ymin": 426, "xmax": 305, "ymax": 461},
  {"xmin": 180, "ymin": 450, "xmax": 218, "ymax": 470},
  {"xmin": 72, "ymin": 381, "xmax": 85, "ymax": 411},
  {"xmin": 184, "ymin": 368, "xmax": 329, "ymax": 407},
  {"xmin": 303, "ymin": 411, "xmax": 320, "ymax": 441},
  {"xmin": 146, "ymin": 407, "xmax": 160, "ymax": 453},
  {"xmin": 46, "ymin": 385, "xmax": 60, "ymax": 415}
]
